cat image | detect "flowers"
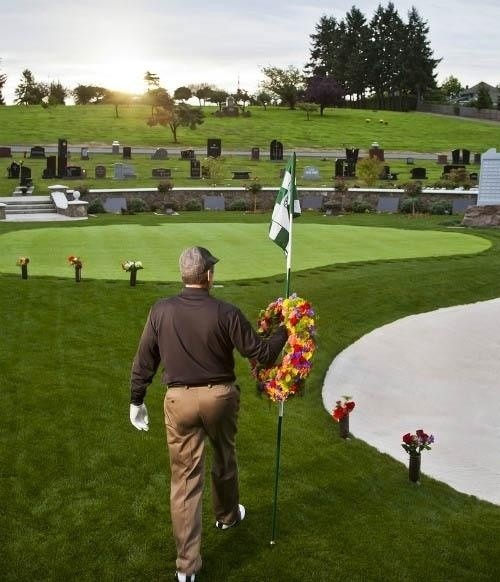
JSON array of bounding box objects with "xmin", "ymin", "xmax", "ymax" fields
[
  {"xmin": 16, "ymin": 256, "xmax": 30, "ymax": 266},
  {"xmin": 332, "ymin": 395, "xmax": 355, "ymax": 421},
  {"xmin": 66, "ymin": 255, "xmax": 84, "ymax": 269},
  {"xmin": 248, "ymin": 294, "xmax": 320, "ymax": 412},
  {"xmin": 400, "ymin": 429, "xmax": 435, "ymax": 454},
  {"xmin": 121, "ymin": 258, "xmax": 145, "ymax": 273}
]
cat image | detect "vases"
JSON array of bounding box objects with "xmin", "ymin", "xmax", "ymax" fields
[
  {"xmin": 339, "ymin": 416, "xmax": 349, "ymax": 438},
  {"xmin": 409, "ymin": 453, "xmax": 421, "ymax": 482},
  {"xmin": 130, "ymin": 269, "xmax": 137, "ymax": 287},
  {"xmin": 20, "ymin": 265, "xmax": 28, "ymax": 279},
  {"xmin": 75, "ymin": 267, "xmax": 81, "ymax": 282}
]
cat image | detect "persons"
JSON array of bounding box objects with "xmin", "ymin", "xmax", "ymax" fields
[{"xmin": 129, "ymin": 245, "xmax": 288, "ymax": 582}]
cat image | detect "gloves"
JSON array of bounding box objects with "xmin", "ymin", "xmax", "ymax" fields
[{"xmin": 130, "ymin": 404, "xmax": 149, "ymax": 432}]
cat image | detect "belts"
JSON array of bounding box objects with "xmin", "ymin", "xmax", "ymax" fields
[{"xmin": 168, "ymin": 382, "xmax": 224, "ymax": 387}]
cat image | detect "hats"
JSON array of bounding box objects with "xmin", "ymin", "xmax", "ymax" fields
[{"xmin": 179, "ymin": 246, "xmax": 219, "ymax": 277}]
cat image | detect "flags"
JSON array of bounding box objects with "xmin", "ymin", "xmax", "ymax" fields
[{"xmin": 269, "ymin": 152, "xmax": 302, "ymax": 256}]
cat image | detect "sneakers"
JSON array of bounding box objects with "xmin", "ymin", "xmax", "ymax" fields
[
  {"xmin": 216, "ymin": 504, "xmax": 245, "ymax": 529},
  {"xmin": 175, "ymin": 570, "xmax": 196, "ymax": 582}
]
